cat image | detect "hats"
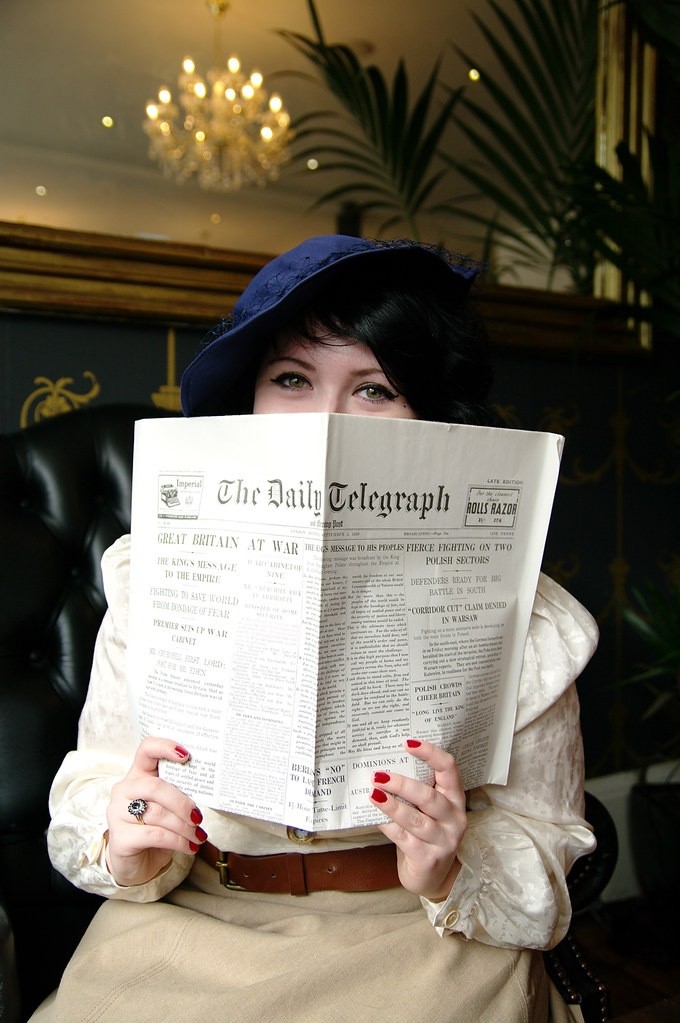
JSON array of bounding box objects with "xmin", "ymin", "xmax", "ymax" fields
[{"xmin": 179, "ymin": 235, "xmax": 495, "ymax": 417}]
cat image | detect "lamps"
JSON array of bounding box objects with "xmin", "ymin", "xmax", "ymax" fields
[{"xmin": 141, "ymin": 0, "xmax": 295, "ymax": 196}]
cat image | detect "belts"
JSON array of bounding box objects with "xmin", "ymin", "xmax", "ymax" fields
[{"xmin": 196, "ymin": 842, "xmax": 403, "ymax": 896}]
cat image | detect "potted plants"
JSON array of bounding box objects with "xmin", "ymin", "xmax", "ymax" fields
[{"xmin": 606, "ymin": 551, "xmax": 680, "ymax": 928}]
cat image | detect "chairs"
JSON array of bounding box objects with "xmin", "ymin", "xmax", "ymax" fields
[{"xmin": 0, "ymin": 403, "xmax": 619, "ymax": 1023}]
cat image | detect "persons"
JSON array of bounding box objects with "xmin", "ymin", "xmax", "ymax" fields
[{"xmin": 27, "ymin": 236, "xmax": 598, "ymax": 1023}]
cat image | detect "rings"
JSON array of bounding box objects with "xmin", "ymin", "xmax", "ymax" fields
[{"xmin": 128, "ymin": 799, "xmax": 148, "ymax": 825}]
